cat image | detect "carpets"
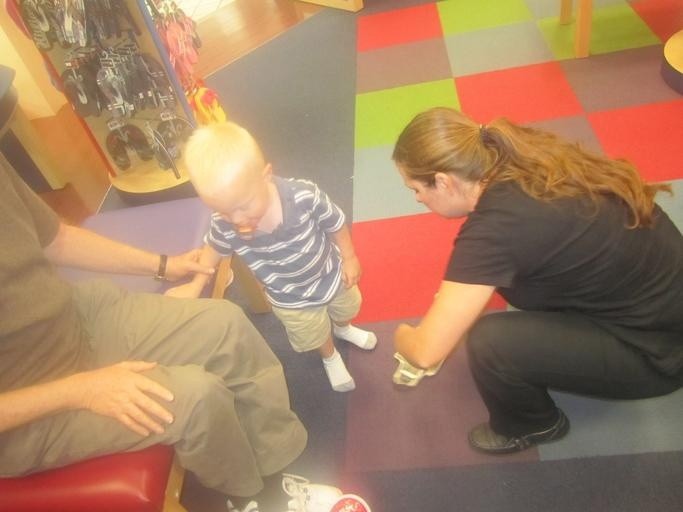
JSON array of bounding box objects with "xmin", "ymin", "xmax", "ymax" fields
[{"xmin": 345, "ymin": 0, "xmax": 683, "ymax": 473}]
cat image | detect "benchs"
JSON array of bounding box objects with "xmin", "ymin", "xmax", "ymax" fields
[{"xmin": 0, "ymin": 197, "xmax": 273, "ymax": 512}]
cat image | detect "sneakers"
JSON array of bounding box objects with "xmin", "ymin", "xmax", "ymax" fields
[
  {"xmin": 228, "ymin": 473, "xmax": 343, "ymax": 512},
  {"xmin": 392, "ymin": 352, "xmax": 444, "ymax": 390},
  {"xmin": 468, "ymin": 407, "xmax": 570, "ymax": 454}
]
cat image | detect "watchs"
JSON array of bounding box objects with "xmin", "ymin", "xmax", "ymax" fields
[{"xmin": 155, "ymin": 256, "xmax": 167, "ymax": 280}]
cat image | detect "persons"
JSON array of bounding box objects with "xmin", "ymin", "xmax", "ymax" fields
[
  {"xmin": 392, "ymin": 107, "xmax": 683, "ymax": 453},
  {"xmin": 0, "ymin": 155, "xmax": 343, "ymax": 512},
  {"xmin": 164, "ymin": 122, "xmax": 378, "ymax": 393}
]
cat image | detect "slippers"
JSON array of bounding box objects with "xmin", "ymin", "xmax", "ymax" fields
[{"xmin": 19, "ymin": 1, "xmax": 226, "ymax": 179}]
